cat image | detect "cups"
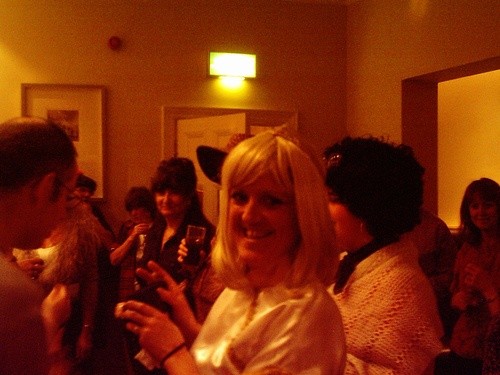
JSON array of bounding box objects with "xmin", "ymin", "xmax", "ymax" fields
[{"xmin": 183, "ymin": 224, "xmax": 208, "ymax": 265}]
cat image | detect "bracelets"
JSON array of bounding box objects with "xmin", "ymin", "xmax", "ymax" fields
[
  {"xmin": 82, "ymin": 325, "xmax": 94, "ymax": 329},
  {"xmin": 159, "ymin": 341, "xmax": 188, "ymax": 367}
]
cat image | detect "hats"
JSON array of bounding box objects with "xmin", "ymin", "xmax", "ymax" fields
[{"xmin": 195, "ymin": 133, "xmax": 258, "ymax": 188}]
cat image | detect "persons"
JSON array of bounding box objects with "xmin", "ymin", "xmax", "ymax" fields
[
  {"xmin": 109, "ymin": 186, "xmax": 157, "ymax": 375},
  {"xmin": 321, "ymin": 135, "xmax": 444, "ymax": 375},
  {"xmin": 0, "ymin": 116, "xmax": 79, "ymax": 375},
  {"xmin": 447, "ymin": 177, "xmax": 500, "ymax": 375},
  {"xmin": 73, "ymin": 171, "xmax": 116, "ymax": 242},
  {"xmin": 120, "ymin": 124, "xmax": 347, "ymax": 375},
  {"xmin": 13, "ymin": 191, "xmax": 100, "ymax": 375},
  {"xmin": 125, "ymin": 157, "xmax": 216, "ymax": 375},
  {"xmin": 177, "ymin": 133, "xmax": 256, "ymax": 326},
  {"xmin": 399, "ymin": 208, "xmax": 457, "ymax": 316}
]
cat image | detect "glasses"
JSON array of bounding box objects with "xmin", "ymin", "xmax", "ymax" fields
[
  {"xmin": 324, "ymin": 152, "xmax": 343, "ymax": 171},
  {"xmin": 55, "ymin": 177, "xmax": 86, "ymax": 211}
]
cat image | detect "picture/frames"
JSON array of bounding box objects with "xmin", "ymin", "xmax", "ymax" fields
[{"xmin": 20, "ymin": 83, "xmax": 108, "ymax": 203}]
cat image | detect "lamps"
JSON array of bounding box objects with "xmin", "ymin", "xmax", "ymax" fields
[{"xmin": 207, "ymin": 50, "xmax": 257, "ymax": 78}]
